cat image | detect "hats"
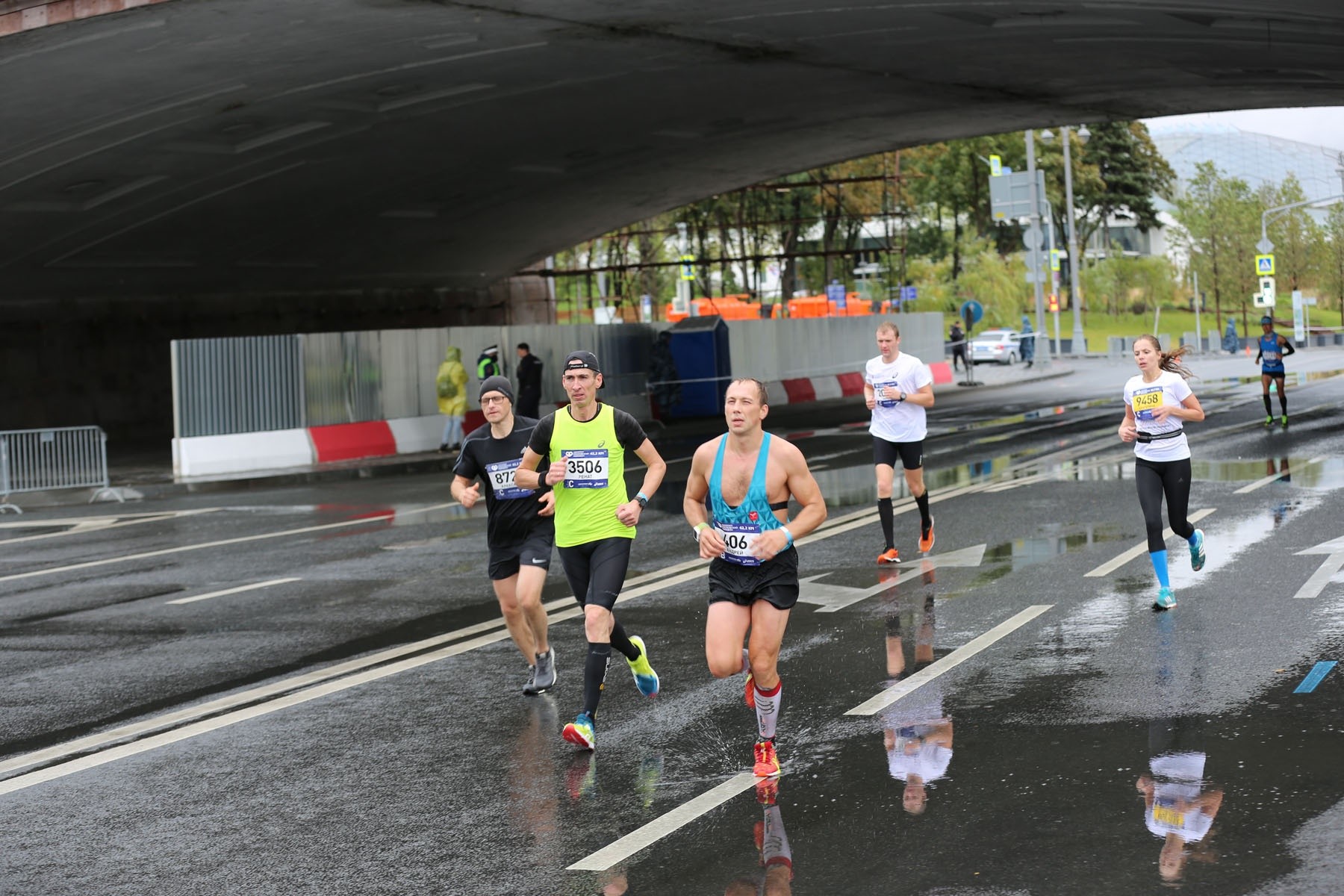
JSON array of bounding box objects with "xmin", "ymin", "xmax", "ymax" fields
[
  {"xmin": 482, "ymin": 345, "xmax": 498, "ymax": 355},
  {"xmin": 1262, "ymin": 318, "xmax": 1271, "ymax": 324},
  {"xmin": 563, "ymin": 351, "xmax": 605, "ymax": 389},
  {"xmin": 478, "ymin": 375, "xmax": 515, "ymax": 405}
]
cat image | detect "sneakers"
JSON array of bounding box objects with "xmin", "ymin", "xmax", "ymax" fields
[
  {"xmin": 562, "ymin": 713, "xmax": 595, "ymax": 749},
  {"xmin": 523, "ymin": 664, "xmax": 546, "ymax": 696},
  {"xmin": 756, "ymin": 777, "xmax": 780, "ymax": 803},
  {"xmin": 532, "ymin": 646, "xmax": 556, "ymax": 689},
  {"xmin": 745, "ymin": 668, "xmax": 755, "ymax": 708},
  {"xmin": 1188, "ymin": 529, "xmax": 1206, "ymax": 571},
  {"xmin": 918, "ymin": 514, "xmax": 935, "ymax": 552},
  {"xmin": 625, "ymin": 635, "xmax": 660, "ymax": 696},
  {"xmin": 878, "ymin": 548, "xmax": 901, "ymax": 563},
  {"xmin": 1282, "ymin": 421, "xmax": 1288, "ymax": 428},
  {"xmin": 753, "ymin": 820, "xmax": 764, "ymax": 850},
  {"xmin": 1151, "ymin": 587, "xmax": 1177, "ymax": 609},
  {"xmin": 1265, "ymin": 419, "xmax": 1275, "ymax": 427},
  {"xmin": 753, "ymin": 739, "xmax": 781, "ymax": 777}
]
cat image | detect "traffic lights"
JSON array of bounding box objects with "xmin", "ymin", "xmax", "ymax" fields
[
  {"xmin": 1048, "ymin": 294, "xmax": 1060, "ymax": 312},
  {"xmin": 1254, "ymin": 275, "xmax": 1277, "ymax": 308}
]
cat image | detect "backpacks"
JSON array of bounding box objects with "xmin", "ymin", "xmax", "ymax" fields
[{"xmin": 436, "ymin": 361, "xmax": 461, "ymax": 399}]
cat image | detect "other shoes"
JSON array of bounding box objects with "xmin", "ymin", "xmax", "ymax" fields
[
  {"xmin": 438, "ymin": 443, "xmax": 451, "ymax": 454},
  {"xmin": 1025, "ymin": 361, "xmax": 1033, "ymax": 368},
  {"xmin": 450, "ymin": 443, "xmax": 461, "ymax": 453}
]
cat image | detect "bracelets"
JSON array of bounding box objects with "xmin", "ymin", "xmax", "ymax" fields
[
  {"xmin": 636, "ymin": 492, "xmax": 648, "ymax": 503},
  {"xmin": 538, "ymin": 471, "xmax": 550, "ymax": 489},
  {"xmin": 779, "ymin": 526, "xmax": 792, "ymax": 542}
]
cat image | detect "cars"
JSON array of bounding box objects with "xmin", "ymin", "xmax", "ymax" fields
[{"xmin": 966, "ymin": 328, "xmax": 1022, "ymax": 367}]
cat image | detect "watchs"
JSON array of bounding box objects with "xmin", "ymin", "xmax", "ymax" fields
[
  {"xmin": 899, "ymin": 392, "xmax": 906, "ymax": 401},
  {"xmin": 693, "ymin": 522, "xmax": 710, "ymax": 542},
  {"xmin": 632, "ymin": 497, "xmax": 647, "ymax": 508}
]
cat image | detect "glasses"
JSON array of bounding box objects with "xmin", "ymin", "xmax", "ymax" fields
[{"xmin": 478, "ymin": 396, "xmax": 506, "ymax": 406}]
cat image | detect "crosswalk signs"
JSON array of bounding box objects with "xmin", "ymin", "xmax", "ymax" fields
[
  {"xmin": 1255, "ymin": 254, "xmax": 1276, "ymax": 276},
  {"xmin": 680, "ymin": 254, "xmax": 698, "ymax": 281}
]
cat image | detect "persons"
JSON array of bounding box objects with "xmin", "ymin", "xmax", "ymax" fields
[
  {"xmin": 684, "ymin": 378, "xmax": 827, "ymax": 776},
  {"xmin": 435, "ymin": 347, "xmax": 470, "ymax": 454},
  {"xmin": 476, "ymin": 343, "xmax": 501, "ymax": 384},
  {"xmin": 515, "ymin": 343, "xmax": 543, "ymax": 420},
  {"xmin": 1117, "ymin": 335, "xmax": 1206, "ymax": 610},
  {"xmin": 451, "ymin": 377, "xmax": 556, "ymax": 695},
  {"xmin": 1255, "ymin": 316, "xmax": 1295, "ymax": 428},
  {"xmin": 1222, "ymin": 318, "xmax": 1240, "ymax": 355},
  {"xmin": 1136, "ymin": 613, "xmax": 1230, "ymax": 892},
  {"xmin": 638, "ymin": 331, "xmax": 680, "ymax": 424},
  {"xmin": 1019, "ymin": 316, "xmax": 1036, "ymax": 368},
  {"xmin": 724, "ymin": 776, "xmax": 793, "ymax": 896},
  {"xmin": 948, "ymin": 320, "xmax": 973, "ymax": 373},
  {"xmin": 514, "ymin": 350, "xmax": 667, "ymax": 749},
  {"xmin": 864, "ymin": 322, "xmax": 935, "ymax": 563},
  {"xmin": 877, "ymin": 562, "xmax": 952, "ymax": 817}
]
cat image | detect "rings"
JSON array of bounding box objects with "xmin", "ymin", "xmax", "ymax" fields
[
  {"xmin": 1128, "ymin": 437, "xmax": 1130, "ymax": 440},
  {"xmin": 870, "ymin": 406, "xmax": 872, "ymax": 408}
]
